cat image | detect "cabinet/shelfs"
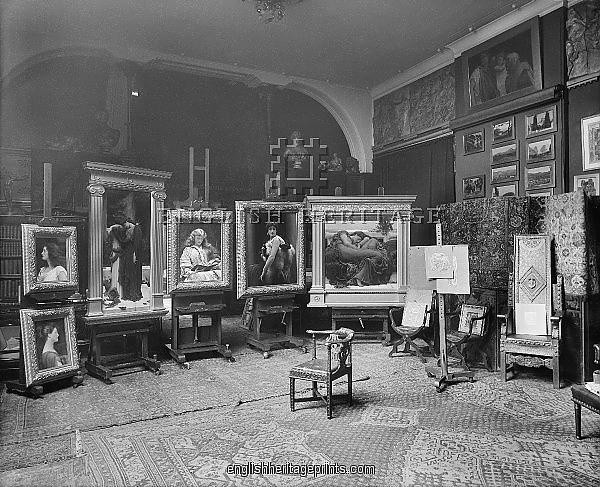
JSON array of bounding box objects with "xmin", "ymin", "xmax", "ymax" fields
[{"xmin": 1, "ymin": 236, "xmax": 23, "ymax": 315}]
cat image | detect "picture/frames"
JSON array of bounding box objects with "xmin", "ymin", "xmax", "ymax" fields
[
  {"xmin": 20, "ymin": 305, "xmax": 81, "ymax": 387},
  {"xmin": 457, "ymin": 305, "xmax": 487, "ymax": 335},
  {"xmin": 235, "ymin": 200, "xmax": 309, "ymax": 301},
  {"xmin": 166, "ymin": 209, "xmax": 234, "ymax": 294},
  {"xmin": 574, "ymin": 172, "xmax": 600, "ymax": 197},
  {"xmin": 20, "ymin": 223, "xmax": 80, "ymax": 292},
  {"xmin": 580, "ymin": 113, "xmax": 600, "ymax": 171},
  {"xmin": 462, "ymin": 104, "xmax": 559, "ymax": 201},
  {"xmin": 460, "ymin": 15, "xmax": 544, "ymax": 117}
]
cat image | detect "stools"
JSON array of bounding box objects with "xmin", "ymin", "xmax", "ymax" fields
[{"xmin": 570, "ymin": 382, "xmax": 600, "ymax": 439}]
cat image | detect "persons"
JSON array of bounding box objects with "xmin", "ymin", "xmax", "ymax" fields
[
  {"xmin": 39, "ymin": 325, "xmax": 61, "ymax": 368},
  {"xmin": 107, "ymin": 215, "xmax": 141, "ymax": 302},
  {"xmin": 332, "ymin": 231, "xmax": 387, "ymax": 287},
  {"xmin": 180, "ymin": 228, "xmax": 221, "ymax": 279},
  {"xmin": 260, "ymin": 221, "xmax": 291, "ymax": 284},
  {"xmin": 469, "ymin": 54, "xmax": 534, "ymax": 106},
  {"xmin": 36, "ymin": 243, "xmax": 67, "ymax": 283}
]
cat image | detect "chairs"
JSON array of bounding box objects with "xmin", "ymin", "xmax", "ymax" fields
[
  {"xmin": 498, "ymin": 271, "xmax": 565, "ymax": 390},
  {"xmin": 388, "ymin": 293, "xmax": 435, "ymax": 363},
  {"xmin": 432, "ymin": 302, "xmax": 490, "ymax": 372},
  {"xmin": 289, "ymin": 326, "xmax": 355, "ymax": 419}
]
